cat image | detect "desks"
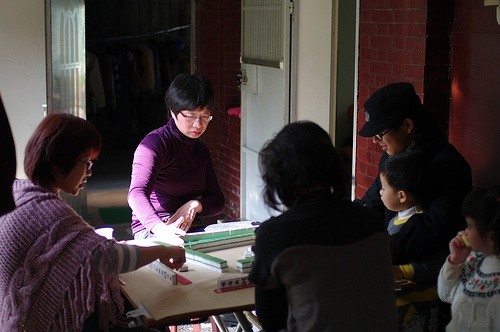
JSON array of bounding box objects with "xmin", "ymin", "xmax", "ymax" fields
[{"xmin": 118, "ymin": 230, "xmax": 255, "ymax": 332}]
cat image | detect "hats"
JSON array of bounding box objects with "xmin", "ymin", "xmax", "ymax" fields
[{"xmin": 359, "ymin": 82, "xmax": 421, "ymax": 135}]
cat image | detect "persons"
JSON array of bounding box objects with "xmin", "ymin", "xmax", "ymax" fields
[
  {"xmin": 379, "ymin": 152, "xmax": 439, "ymax": 330},
  {"xmin": 346, "ymin": 83, "xmax": 472, "ymax": 332},
  {"xmin": 248, "ymin": 123, "xmax": 399, "ymax": 332},
  {"xmin": 127, "ymin": 74, "xmax": 225, "ymax": 246},
  {"xmin": 437, "ymin": 186, "xmax": 500, "ymax": 332},
  {"xmin": 1, "ymin": 114, "xmax": 185, "ymax": 332}
]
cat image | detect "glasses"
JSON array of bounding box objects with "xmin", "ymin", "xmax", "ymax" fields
[
  {"xmin": 79, "ymin": 160, "xmax": 93, "ymax": 170},
  {"xmin": 376, "ymin": 129, "xmax": 392, "ymax": 140},
  {"xmin": 180, "ymin": 112, "xmax": 213, "ymax": 121}
]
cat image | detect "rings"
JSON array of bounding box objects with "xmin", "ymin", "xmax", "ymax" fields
[{"xmin": 183, "ymin": 222, "xmax": 187, "ymax": 226}]
